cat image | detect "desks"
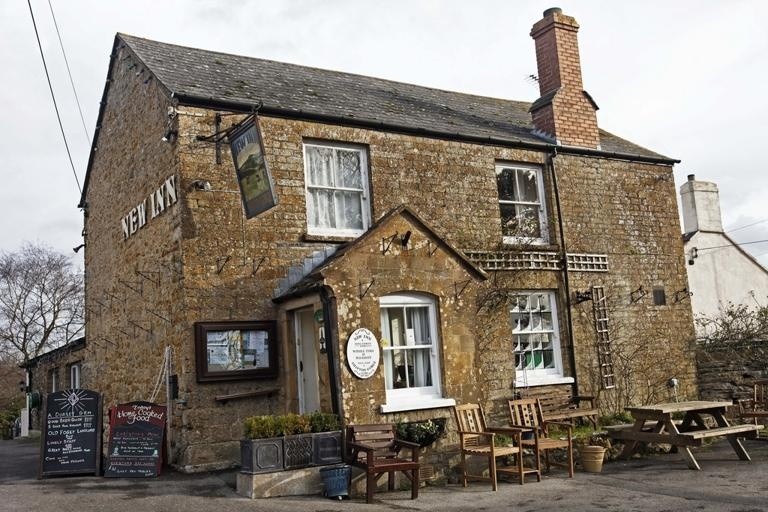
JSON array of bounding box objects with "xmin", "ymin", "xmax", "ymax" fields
[{"xmin": 618, "ymin": 401, "xmax": 751, "ymax": 469}]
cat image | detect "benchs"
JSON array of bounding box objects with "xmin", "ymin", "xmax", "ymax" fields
[
  {"xmin": 602, "ymin": 420, "xmax": 764, "ymax": 470},
  {"xmin": 518, "ymin": 385, "xmax": 599, "ymax": 437}
]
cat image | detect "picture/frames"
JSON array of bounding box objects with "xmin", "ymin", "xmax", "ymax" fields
[{"xmin": 193, "ymin": 320, "xmax": 279, "ymax": 383}]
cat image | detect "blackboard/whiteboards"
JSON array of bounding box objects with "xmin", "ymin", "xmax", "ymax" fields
[
  {"xmin": 103, "ymin": 400, "xmax": 168, "ymax": 478},
  {"xmin": 37, "ymin": 388, "xmax": 103, "ymax": 479}
]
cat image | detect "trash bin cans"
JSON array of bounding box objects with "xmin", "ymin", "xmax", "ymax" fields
[{"xmin": 319, "ymin": 465, "xmax": 351, "ymax": 498}]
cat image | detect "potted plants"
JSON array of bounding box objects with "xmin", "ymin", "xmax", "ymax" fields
[{"xmin": 239, "ymin": 411, "xmax": 344, "ymax": 474}]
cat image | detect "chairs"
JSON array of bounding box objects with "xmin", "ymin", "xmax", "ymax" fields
[
  {"xmin": 454, "ymin": 399, "xmax": 575, "ymax": 492},
  {"xmin": 344, "ymin": 424, "xmax": 421, "ymax": 504},
  {"xmin": 736, "ymin": 380, "xmax": 768, "ymax": 438}
]
